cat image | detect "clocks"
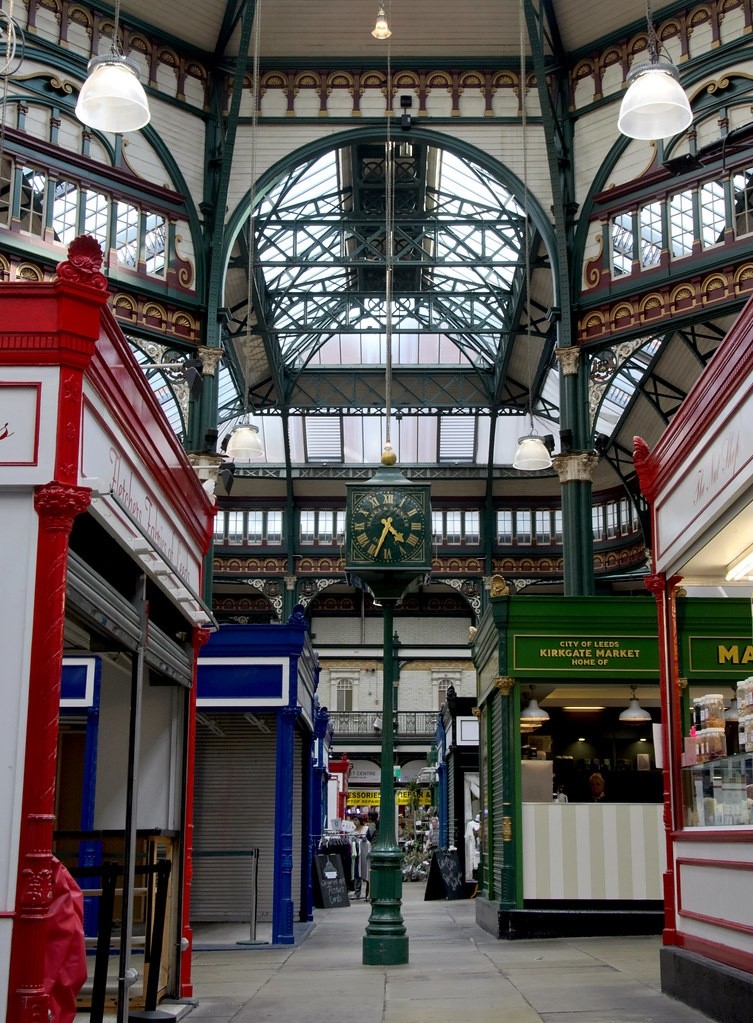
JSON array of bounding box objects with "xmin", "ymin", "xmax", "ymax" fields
[{"xmin": 345, "ymin": 483, "xmax": 431, "ymax": 571}]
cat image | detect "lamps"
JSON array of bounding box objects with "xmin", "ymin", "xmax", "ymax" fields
[
  {"xmin": 617, "ymin": 1, "xmax": 694, "ymax": 141},
  {"xmin": 618, "ymin": 685, "xmax": 652, "ymax": 720},
  {"xmin": 227, "ymin": 1, "xmax": 266, "ymax": 458},
  {"xmin": 220, "ymin": 434, "xmax": 231, "ymax": 453},
  {"xmin": 139, "ymin": 359, "xmax": 205, "ymax": 401},
  {"xmin": 371, "ymin": 2, "xmax": 392, "ymax": 39},
  {"xmin": 204, "ymin": 428, "xmax": 219, "ymax": 453},
  {"xmin": 521, "ymin": 688, "xmax": 551, "ymax": 733},
  {"xmin": 513, "ymin": 0, "xmax": 553, "ymax": 472},
  {"xmin": 191, "ymin": 462, "xmax": 235, "ymax": 495},
  {"xmin": 76, "ymin": 0, "xmax": 152, "ymax": 133}
]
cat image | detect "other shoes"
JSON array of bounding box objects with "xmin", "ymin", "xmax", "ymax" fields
[{"xmin": 349, "ymin": 893, "xmax": 361, "ymax": 900}]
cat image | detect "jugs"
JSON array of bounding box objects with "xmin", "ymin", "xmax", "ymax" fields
[{"xmin": 557, "ymin": 792, "xmax": 568, "ymax": 803}]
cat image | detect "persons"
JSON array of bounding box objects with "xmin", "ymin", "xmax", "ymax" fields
[
  {"xmin": 398, "ymin": 817, "xmax": 440, "ymax": 858},
  {"xmin": 464, "ymin": 814, "xmax": 481, "ymax": 898},
  {"xmin": 350, "ymin": 810, "xmax": 380, "ymax": 901},
  {"xmin": 581, "ymin": 770, "xmax": 618, "ymax": 803}
]
